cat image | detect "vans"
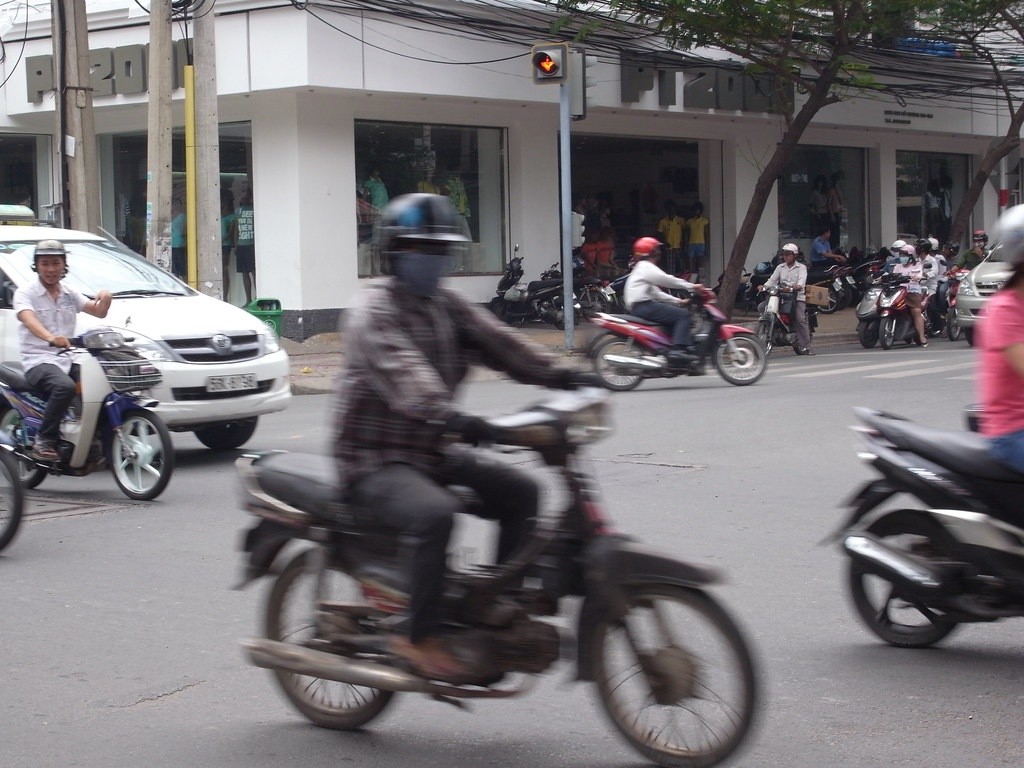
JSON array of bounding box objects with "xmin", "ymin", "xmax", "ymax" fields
[{"xmin": 954, "ymin": 202, "xmax": 1024, "ymax": 348}]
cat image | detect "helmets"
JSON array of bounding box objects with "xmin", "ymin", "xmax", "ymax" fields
[
  {"xmin": 915, "ymin": 239, "xmax": 932, "ymax": 253},
  {"xmin": 633, "ymin": 237, "xmax": 663, "ymax": 256},
  {"xmin": 377, "ymin": 193, "xmax": 469, "ymax": 256},
  {"xmin": 781, "ymin": 243, "xmax": 798, "ymax": 255},
  {"xmin": 972, "ymin": 230, "xmax": 987, "ymax": 248},
  {"xmin": 945, "ymin": 240, "xmax": 959, "ymax": 253},
  {"xmin": 927, "ymin": 237, "xmax": 939, "ymax": 250},
  {"xmin": 900, "ymin": 245, "xmax": 915, "ymax": 257},
  {"xmin": 998, "ymin": 204, "xmax": 1024, "ymax": 264},
  {"xmin": 891, "ymin": 240, "xmax": 907, "ymax": 249},
  {"xmin": 33, "ymin": 240, "xmax": 69, "ymax": 268}
]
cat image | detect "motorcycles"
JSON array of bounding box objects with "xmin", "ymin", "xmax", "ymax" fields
[
  {"xmin": 584, "ymin": 279, "xmax": 768, "ymax": 392},
  {"xmin": 716, "ymin": 234, "xmax": 983, "ymax": 351},
  {"xmin": 225, "ymin": 376, "xmax": 759, "ymax": 768},
  {"xmin": 754, "ymin": 281, "xmax": 820, "ymax": 356},
  {"xmin": 815, "ymin": 404, "xmax": 1024, "ymax": 649},
  {"xmin": 483, "ymin": 241, "xmax": 692, "ymax": 330},
  {"xmin": 0, "ymin": 312, "xmax": 177, "ymax": 501}
]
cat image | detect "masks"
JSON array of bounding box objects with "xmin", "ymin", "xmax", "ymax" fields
[
  {"xmin": 899, "ymin": 257, "xmax": 910, "ymax": 264},
  {"xmin": 894, "ymin": 252, "xmax": 900, "ymax": 259}
]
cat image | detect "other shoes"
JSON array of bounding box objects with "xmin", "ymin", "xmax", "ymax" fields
[
  {"xmin": 668, "ymin": 351, "xmax": 697, "ymax": 361},
  {"xmin": 31, "ymin": 443, "xmax": 59, "ymax": 461},
  {"xmin": 388, "ymin": 633, "xmax": 460, "ymax": 680},
  {"xmin": 807, "ymin": 347, "xmax": 815, "ymax": 356},
  {"xmin": 922, "ymin": 342, "xmax": 927, "ymax": 347}
]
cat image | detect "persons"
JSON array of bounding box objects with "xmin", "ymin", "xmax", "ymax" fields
[
  {"xmin": 221, "ymin": 202, "xmax": 235, "ymax": 301},
  {"xmin": 328, "ymin": 195, "xmax": 590, "ymax": 683},
  {"xmin": 13, "ymin": 239, "xmax": 112, "ymax": 463},
  {"xmin": 657, "ymin": 200, "xmax": 687, "ymax": 276},
  {"xmin": 880, "ymin": 229, "xmax": 990, "ymax": 347},
  {"xmin": 925, "ymin": 175, "xmax": 952, "ymax": 237},
  {"xmin": 979, "ymin": 203, "xmax": 1024, "ymax": 475},
  {"xmin": 810, "ymin": 225, "xmax": 845, "ymax": 265},
  {"xmin": 757, "ymin": 242, "xmax": 815, "ymax": 355},
  {"xmin": 358, "ymin": 167, "xmax": 475, "ymax": 275},
  {"xmin": 171, "ymin": 198, "xmax": 187, "ymax": 282},
  {"xmin": 623, "ymin": 235, "xmax": 707, "ymax": 362},
  {"xmin": 687, "ymin": 201, "xmax": 709, "ymax": 283},
  {"xmin": 575, "ymin": 197, "xmax": 623, "ymax": 278},
  {"xmin": 778, "ymin": 167, "xmax": 842, "ymax": 244},
  {"xmin": 233, "ymin": 187, "xmax": 256, "ymax": 307}
]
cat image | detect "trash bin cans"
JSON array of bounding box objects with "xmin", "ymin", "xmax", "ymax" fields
[{"xmin": 249, "ymin": 298, "xmax": 282, "ymax": 338}]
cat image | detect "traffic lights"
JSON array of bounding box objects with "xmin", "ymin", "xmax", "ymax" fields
[
  {"xmin": 531, "ymin": 42, "xmax": 569, "ymax": 85},
  {"xmin": 569, "ymin": 47, "xmax": 598, "ymax": 122}
]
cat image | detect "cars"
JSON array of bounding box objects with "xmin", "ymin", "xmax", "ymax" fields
[{"xmin": 0, "ymin": 204, "xmax": 296, "ymax": 455}]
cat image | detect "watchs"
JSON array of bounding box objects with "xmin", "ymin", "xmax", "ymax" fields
[{"xmin": 982, "ymin": 254, "xmax": 985, "ymax": 256}]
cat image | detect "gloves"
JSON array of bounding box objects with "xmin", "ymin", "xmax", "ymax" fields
[
  {"xmin": 448, "ymin": 414, "xmax": 490, "ymax": 446},
  {"xmin": 556, "ymin": 365, "xmax": 602, "ymax": 397}
]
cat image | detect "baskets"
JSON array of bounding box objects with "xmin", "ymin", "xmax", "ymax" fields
[{"xmin": 100, "ymin": 358, "xmax": 161, "ymax": 392}]
